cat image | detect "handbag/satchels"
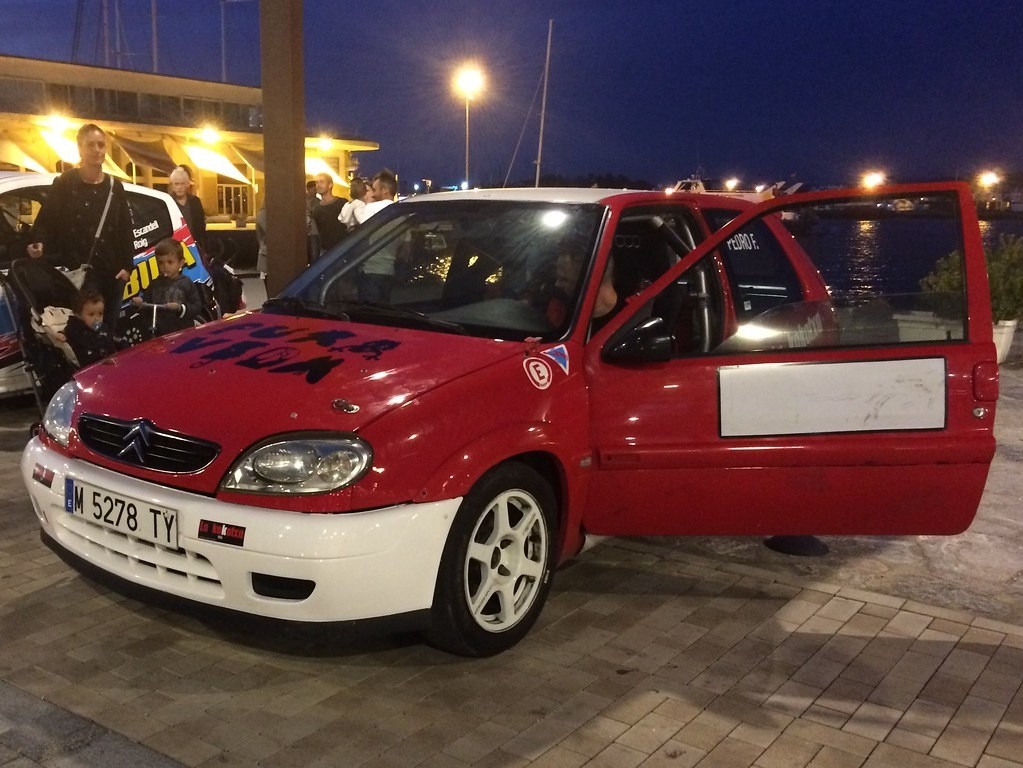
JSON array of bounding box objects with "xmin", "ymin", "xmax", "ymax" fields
[{"xmin": 54, "ymin": 262, "xmax": 90, "ymax": 288}]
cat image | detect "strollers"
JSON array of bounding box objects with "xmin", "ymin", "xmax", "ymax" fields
[{"xmin": 1, "ymin": 253, "xmax": 111, "ymax": 434}]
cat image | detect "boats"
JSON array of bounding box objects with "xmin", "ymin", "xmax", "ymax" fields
[{"xmin": 665, "ymin": 177, "xmax": 803, "ymax": 203}]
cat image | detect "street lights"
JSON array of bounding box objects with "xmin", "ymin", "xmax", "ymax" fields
[{"xmin": 451, "ymin": 64, "xmax": 485, "ymax": 189}]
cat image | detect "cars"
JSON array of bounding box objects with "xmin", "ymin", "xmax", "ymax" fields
[
  {"xmin": 877, "ymin": 199, "xmax": 915, "ymax": 211},
  {"xmin": 0, "ymin": 166, "xmax": 223, "ymax": 400},
  {"xmin": 780, "ymin": 209, "xmax": 799, "ymax": 222},
  {"xmin": 18, "ymin": 182, "xmax": 999, "ymax": 655}
]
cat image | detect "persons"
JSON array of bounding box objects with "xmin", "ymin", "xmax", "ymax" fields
[
  {"xmin": 25, "ymin": 122, "xmax": 133, "ymax": 338},
  {"xmin": 166, "ymin": 165, "xmax": 207, "ymax": 242},
  {"xmin": 553, "ymin": 231, "xmax": 652, "ymax": 362},
  {"xmin": 255, "ymin": 168, "xmax": 399, "ymax": 299},
  {"xmin": 52, "ymin": 289, "xmax": 118, "ymax": 367},
  {"xmin": 130, "ymin": 238, "xmax": 202, "ymax": 334}
]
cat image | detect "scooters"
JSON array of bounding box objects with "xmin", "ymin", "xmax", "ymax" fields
[{"xmin": 131, "ymin": 298, "xmax": 173, "ymax": 340}]
